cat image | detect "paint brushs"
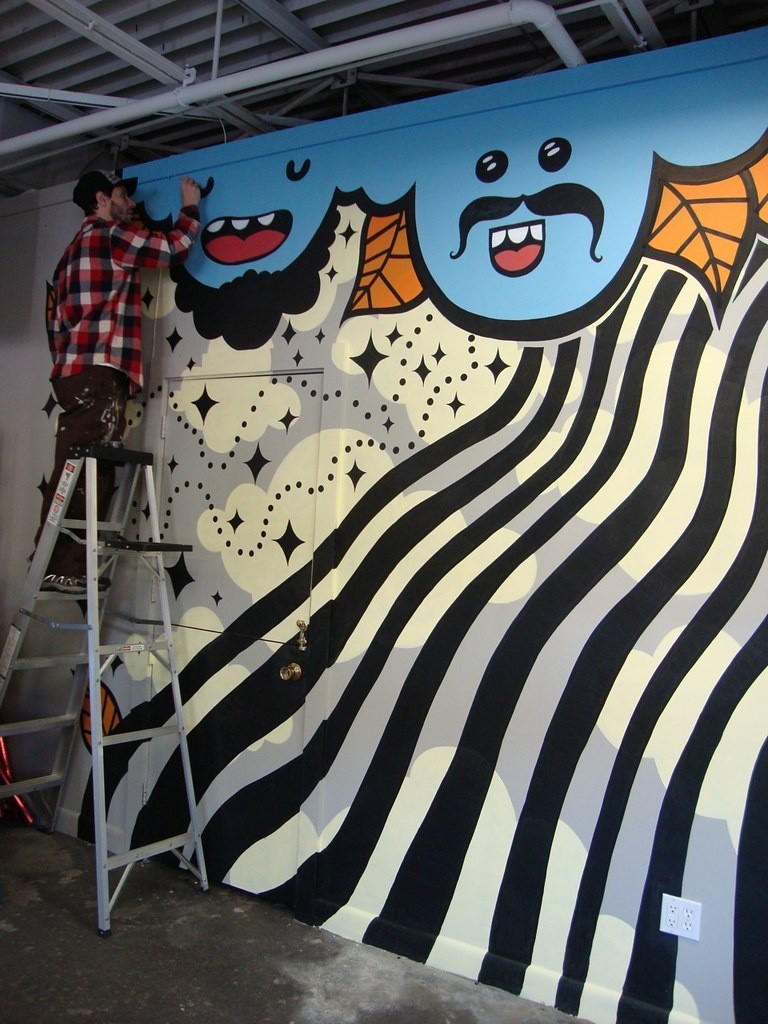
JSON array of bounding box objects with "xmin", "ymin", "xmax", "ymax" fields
[{"xmin": 177, "ymin": 177, "xmax": 183, "ymax": 181}]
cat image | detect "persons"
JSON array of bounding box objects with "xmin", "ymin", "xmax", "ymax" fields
[{"xmin": 27, "ymin": 167, "xmax": 203, "ymax": 602}]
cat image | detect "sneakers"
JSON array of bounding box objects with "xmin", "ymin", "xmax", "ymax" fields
[{"xmin": 37, "ymin": 571, "xmax": 113, "ymax": 595}]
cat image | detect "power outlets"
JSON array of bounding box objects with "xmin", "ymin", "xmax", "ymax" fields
[{"xmin": 659, "ymin": 893, "xmax": 703, "ymax": 942}]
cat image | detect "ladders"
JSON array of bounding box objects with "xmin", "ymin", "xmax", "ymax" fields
[{"xmin": 0, "ymin": 444, "xmax": 212, "ymax": 938}]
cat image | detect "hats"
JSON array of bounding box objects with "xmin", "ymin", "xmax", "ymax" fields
[{"xmin": 71, "ymin": 169, "xmax": 138, "ymax": 210}]
